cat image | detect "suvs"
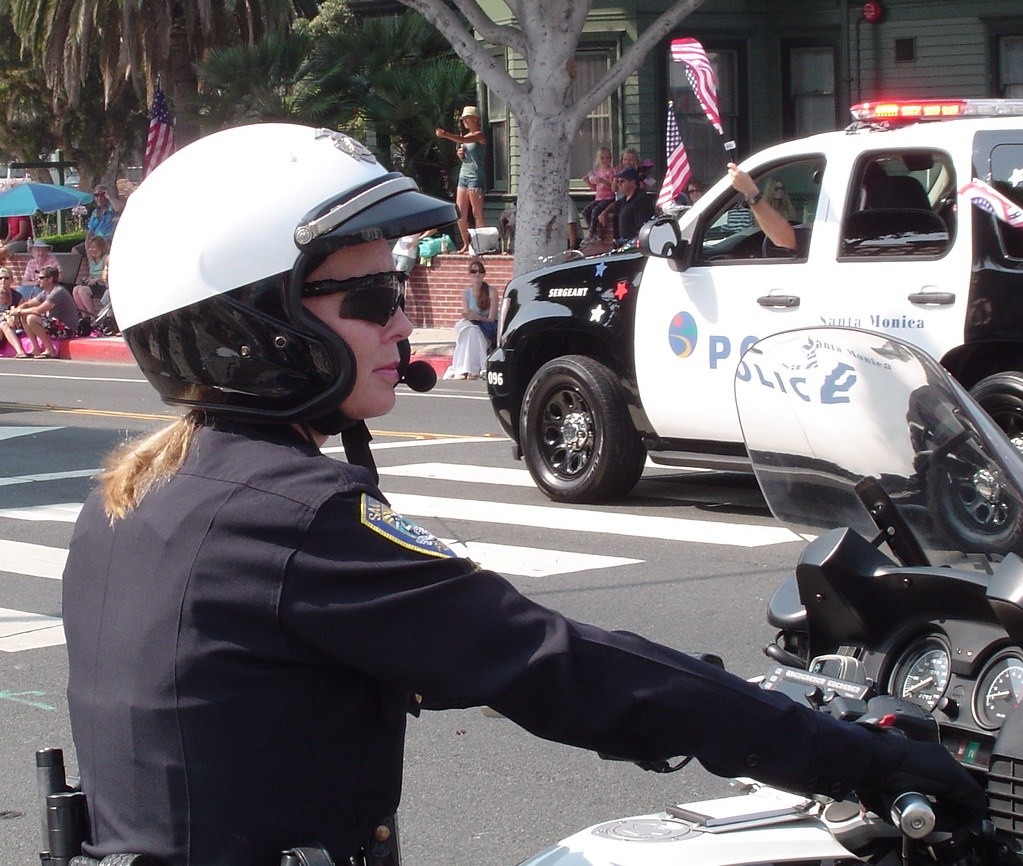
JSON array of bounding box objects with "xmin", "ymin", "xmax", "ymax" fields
[{"xmin": 482, "ymin": 95, "xmax": 1021, "ymax": 513}]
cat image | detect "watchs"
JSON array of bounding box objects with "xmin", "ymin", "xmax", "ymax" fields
[{"xmin": 745, "ymin": 191, "xmax": 763, "ymax": 205}]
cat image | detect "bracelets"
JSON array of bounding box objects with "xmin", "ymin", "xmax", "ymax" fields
[{"xmin": 17, "ymin": 308, "xmax": 22, "ymax": 316}]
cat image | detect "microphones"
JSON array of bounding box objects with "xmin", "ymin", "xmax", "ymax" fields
[{"xmin": 397, "ymin": 361, "xmax": 438, "ymax": 393}]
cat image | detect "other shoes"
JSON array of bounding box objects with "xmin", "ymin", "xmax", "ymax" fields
[{"xmin": 580, "ymin": 235, "xmax": 601, "ymax": 247}]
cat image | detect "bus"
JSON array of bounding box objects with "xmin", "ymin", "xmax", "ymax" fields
[{"xmin": 128, "ymin": 166, "xmax": 143, "ymax": 185}]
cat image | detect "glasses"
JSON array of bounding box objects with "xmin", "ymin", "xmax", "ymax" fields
[
  {"xmin": 617, "ymin": 180, "xmax": 623, "ymax": 183},
  {"xmin": 470, "ymin": 269, "xmax": 486, "ymax": 274},
  {"xmin": 301, "ymin": 270, "xmax": 409, "ymax": 327},
  {"xmin": 0, "ymin": 277, "xmax": 9, "ymax": 280},
  {"xmin": 39, "ymin": 276, "xmax": 44, "ymax": 279},
  {"xmin": 94, "ymin": 193, "xmax": 103, "ymax": 196},
  {"xmin": 688, "ymin": 189, "xmax": 700, "ymax": 193},
  {"xmin": 774, "ymin": 187, "xmax": 784, "ymax": 191}
]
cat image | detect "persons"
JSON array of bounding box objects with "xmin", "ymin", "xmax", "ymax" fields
[
  {"xmin": 756, "ymin": 174, "xmax": 800, "ymax": 225},
  {"xmin": 686, "ymin": 179, "xmax": 706, "ymax": 203},
  {"xmin": 567, "ymin": 145, "xmax": 655, "ymax": 252},
  {"xmin": 0, "ymin": 216, "xmax": 33, "ymax": 265},
  {"xmin": 452, "ymin": 261, "xmax": 498, "ymax": 381},
  {"xmin": 393, "ymin": 228, "xmax": 437, "ymax": 275},
  {"xmin": 72, "ymin": 236, "xmax": 114, "ymax": 334},
  {"xmin": 0, "ymin": 239, "xmax": 79, "ymax": 359},
  {"xmin": 71, "ymin": 185, "xmax": 124, "ymax": 256},
  {"xmin": 727, "ymin": 162, "xmax": 813, "ymax": 259},
  {"xmin": 436, "ymin": 106, "xmax": 487, "ymax": 254},
  {"xmin": 61, "ymin": 124, "xmax": 990, "ymax": 866}
]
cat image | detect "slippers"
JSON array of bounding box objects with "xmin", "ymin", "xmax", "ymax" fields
[
  {"xmin": 18, "ymin": 353, "xmax": 34, "ymax": 358},
  {"xmin": 35, "ymin": 354, "xmax": 60, "ymax": 359}
]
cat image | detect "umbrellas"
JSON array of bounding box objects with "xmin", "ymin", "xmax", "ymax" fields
[{"xmin": 0, "ymin": 183, "xmax": 95, "ymax": 240}]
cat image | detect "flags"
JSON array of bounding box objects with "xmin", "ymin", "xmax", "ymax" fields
[
  {"xmin": 655, "ymin": 36, "xmax": 724, "ymax": 207},
  {"xmin": 141, "ymin": 78, "xmax": 177, "ymax": 182}
]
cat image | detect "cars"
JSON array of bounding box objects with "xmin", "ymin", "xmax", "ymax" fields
[{"xmin": 65, "ymin": 176, "xmax": 80, "ymax": 189}]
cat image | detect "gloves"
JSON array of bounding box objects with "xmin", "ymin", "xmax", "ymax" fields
[{"xmin": 853, "ymin": 731, "xmax": 989, "ymax": 864}]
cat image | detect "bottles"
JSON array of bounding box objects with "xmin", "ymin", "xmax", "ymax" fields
[{"xmin": 27, "ymin": 237, "xmax": 32, "ymax": 253}]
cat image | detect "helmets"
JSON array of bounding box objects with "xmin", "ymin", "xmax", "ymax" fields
[{"xmin": 108, "ymin": 123, "xmax": 462, "ymax": 426}]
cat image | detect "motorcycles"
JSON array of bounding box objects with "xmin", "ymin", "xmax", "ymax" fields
[{"xmin": 511, "ymin": 325, "xmax": 1022, "ymax": 866}]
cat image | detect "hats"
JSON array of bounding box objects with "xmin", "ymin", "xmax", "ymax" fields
[
  {"xmin": 640, "ymin": 159, "xmax": 657, "ymax": 166},
  {"xmin": 460, "ymin": 106, "xmax": 480, "ymax": 120},
  {"xmin": 614, "ymin": 167, "xmax": 641, "ymax": 180},
  {"xmin": 28, "ymin": 240, "xmax": 53, "ymax": 252}
]
